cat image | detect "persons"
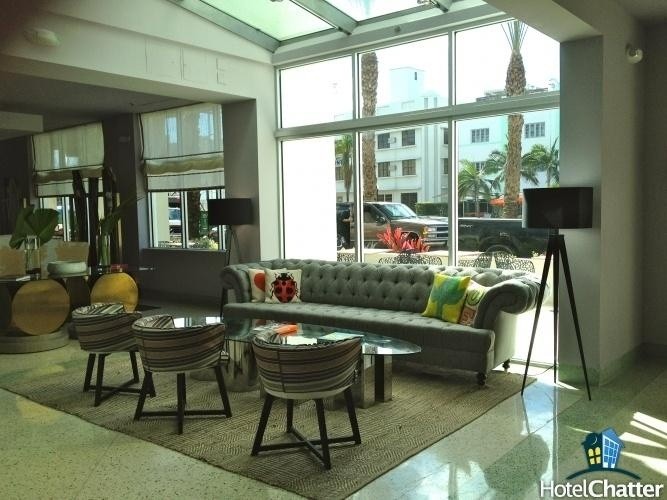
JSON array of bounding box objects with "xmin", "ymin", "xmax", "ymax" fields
[{"xmin": 340, "ymin": 207, "xmax": 353, "ymax": 248}]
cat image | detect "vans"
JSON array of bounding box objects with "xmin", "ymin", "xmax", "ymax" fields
[{"xmin": 168, "ymin": 207, "xmax": 181, "ymax": 233}]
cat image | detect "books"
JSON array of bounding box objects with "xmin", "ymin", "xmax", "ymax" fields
[
  {"xmin": 316, "ymin": 331, "xmax": 364, "ymax": 345},
  {"xmin": 111, "ymin": 263, "xmax": 128, "ymax": 271}
]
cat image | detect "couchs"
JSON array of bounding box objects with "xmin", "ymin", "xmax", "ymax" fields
[{"xmin": 220, "ymin": 259, "xmax": 541, "ymax": 386}]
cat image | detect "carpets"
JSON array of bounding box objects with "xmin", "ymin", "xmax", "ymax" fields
[{"xmin": 0, "ymin": 352, "xmax": 537, "ymax": 500}]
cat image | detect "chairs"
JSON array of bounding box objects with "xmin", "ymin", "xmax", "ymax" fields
[
  {"xmin": 72, "ymin": 302, "xmax": 156, "ymax": 406},
  {"xmin": 250, "ymin": 330, "xmax": 362, "ymax": 470},
  {"xmin": 131, "ymin": 314, "xmax": 232, "ymax": 435}
]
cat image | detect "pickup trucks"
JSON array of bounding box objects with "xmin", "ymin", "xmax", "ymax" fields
[{"xmin": 336, "ymin": 202, "xmax": 550, "ymax": 257}]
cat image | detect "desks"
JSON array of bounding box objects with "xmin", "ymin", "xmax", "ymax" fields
[
  {"xmin": 87, "ymin": 265, "xmax": 157, "ymax": 310},
  {"xmin": 0, "ymin": 269, "xmax": 99, "ymax": 353}
]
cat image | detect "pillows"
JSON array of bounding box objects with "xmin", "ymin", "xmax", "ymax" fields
[
  {"xmin": 264, "ymin": 268, "xmax": 302, "ymax": 303},
  {"xmin": 421, "ymin": 274, "xmax": 471, "ymax": 324},
  {"xmin": 248, "ymin": 268, "xmax": 287, "ymax": 303},
  {"xmin": 459, "ymin": 279, "xmax": 492, "ymax": 327}
]
